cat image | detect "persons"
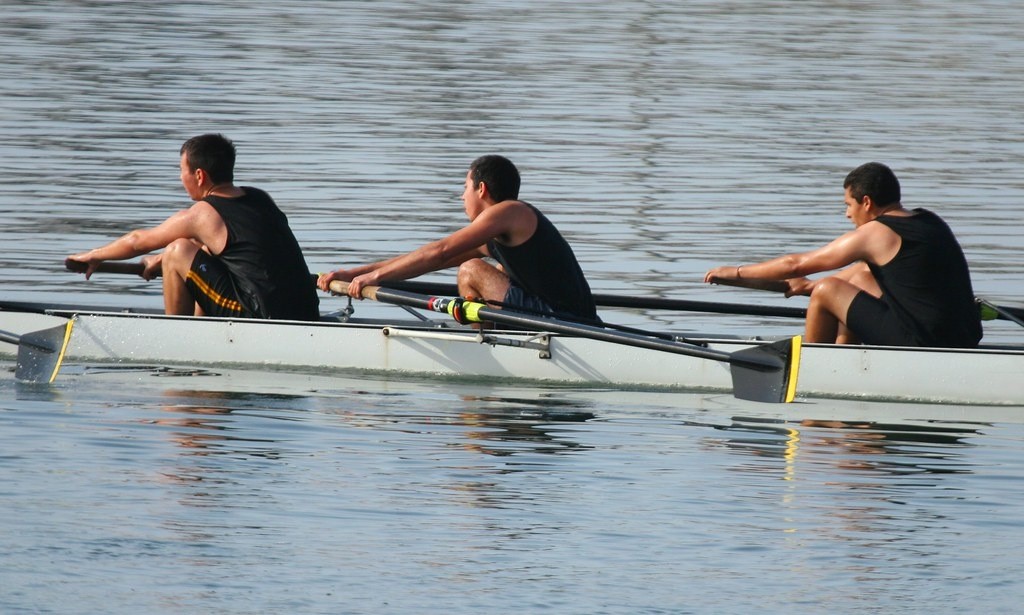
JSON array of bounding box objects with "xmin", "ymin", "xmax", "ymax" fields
[
  {"xmin": 314, "ymin": 154, "xmax": 606, "ymax": 335},
  {"xmin": 65, "ymin": 133, "xmax": 322, "ymax": 321},
  {"xmin": 701, "ymin": 162, "xmax": 985, "ymax": 350}
]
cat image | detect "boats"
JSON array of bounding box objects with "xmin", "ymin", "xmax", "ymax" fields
[
  {"xmin": 675, "ymin": 324, "xmax": 1023, "ymax": 408},
  {"xmin": 1, "ymin": 250, "xmax": 797, "ymax": 403},
  {"xmin": 1, "ymin": 313, "xmax": 67, "ymax": 382}
]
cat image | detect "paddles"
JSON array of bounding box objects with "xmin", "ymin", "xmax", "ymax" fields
[
  {"xmin": 0, "ymin": 318, "xmax": 74, "ymax": 384},
  {"xmin": 709, "ymin": 274, "xmax": 1024, "ymax": 321},
  {"xmin": 65, "ymin": 254, "xmax": 811, "ymax": 319},
  {"xmin": 329, "ymin": 279, "xmax": 803, "ymax": 404}
]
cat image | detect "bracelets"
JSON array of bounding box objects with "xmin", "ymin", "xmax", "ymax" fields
[{"xmin": 737, "ymin": 266, "xmax": 744, "ymax": 281}]
